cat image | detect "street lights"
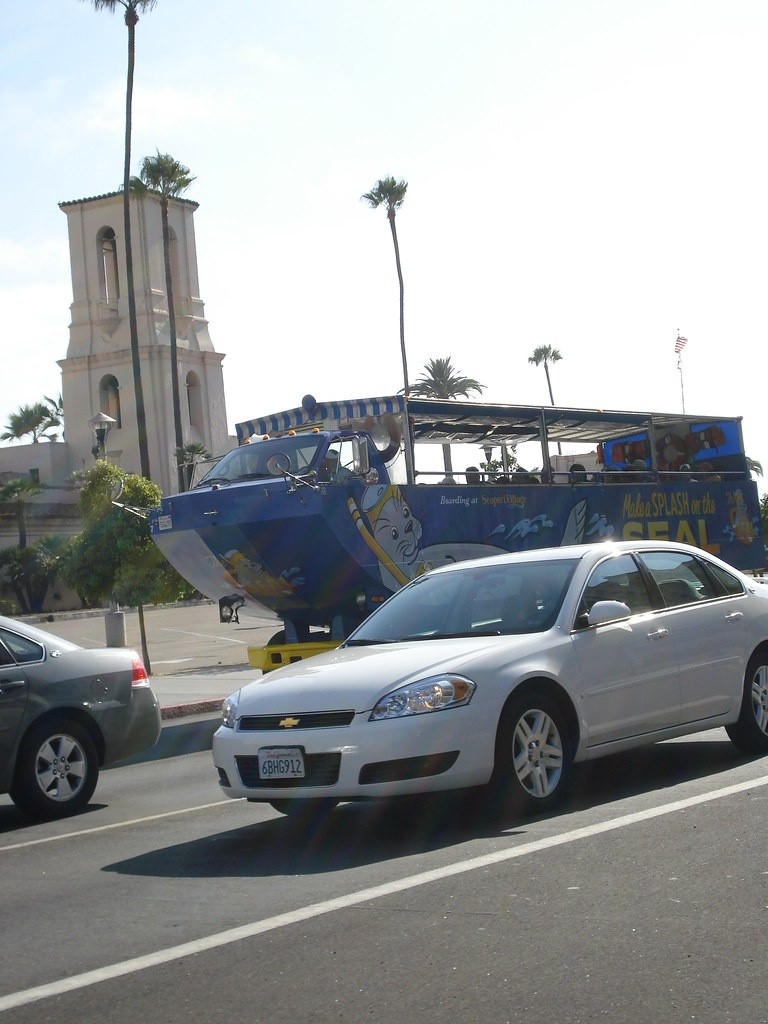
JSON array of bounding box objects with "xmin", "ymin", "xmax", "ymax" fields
[{"xmin": 86, "ymin": 411, "xmax": 127, "ymax": 648}]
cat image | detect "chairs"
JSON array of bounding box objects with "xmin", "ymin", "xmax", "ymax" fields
[
  {"xmin": 657, "ymin": 579, "xmax": 704, "ymax": 606},
  {"xmin": 589, "ymin": 582, "xmax": 628, "ymax": 611}
]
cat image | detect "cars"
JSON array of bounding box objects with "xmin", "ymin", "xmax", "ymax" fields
[
  {"xmin": 212, "ymin": 540, "xmax": 768, "ymax": 817},
  {"xmin": 0, "ymin": 614, "xmax": 162, "ymax": 820}
]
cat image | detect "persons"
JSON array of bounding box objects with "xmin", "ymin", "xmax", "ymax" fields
[
  {"xmin": 466, "ymin": 467, "xmax": 491, "ymax": 484},
  {"xmin": 324, "ymin": 449, "xmax": 353, "ymax": 479},
  {"xmin": 678, "ymin": 463, "xmax": 701, "ymax": 482},
  {"xmin": 569, "ymin": 464, "xmax": 591, "ymax": 482},
  {"xmin": 604, "ymin": 457, "xmax": 648, "ymax": 483},
  {"xmin": 496, "ymin": 467, "xmax": 540, "ymax": 484},
  {"xmin": 700, "ymin": 462, "xmax": 721, "ymax": 482}
]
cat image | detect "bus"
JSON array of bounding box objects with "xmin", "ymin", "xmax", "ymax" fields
[{"xmin": 111, "ymin": 395, "xmax": 767, "ymax": 675}]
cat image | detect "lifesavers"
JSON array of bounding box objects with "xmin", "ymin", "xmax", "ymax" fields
[
  {"xmin": 655, "ymin": 434, "xmax": 688, "ymax": 469},
  {"xmin": 361, "ymin": 413, "xmax": 402, "ymax": 463},
  {"xmin": 596, "ymin": 444, "xmax": 603, "ymax": 464}
]
